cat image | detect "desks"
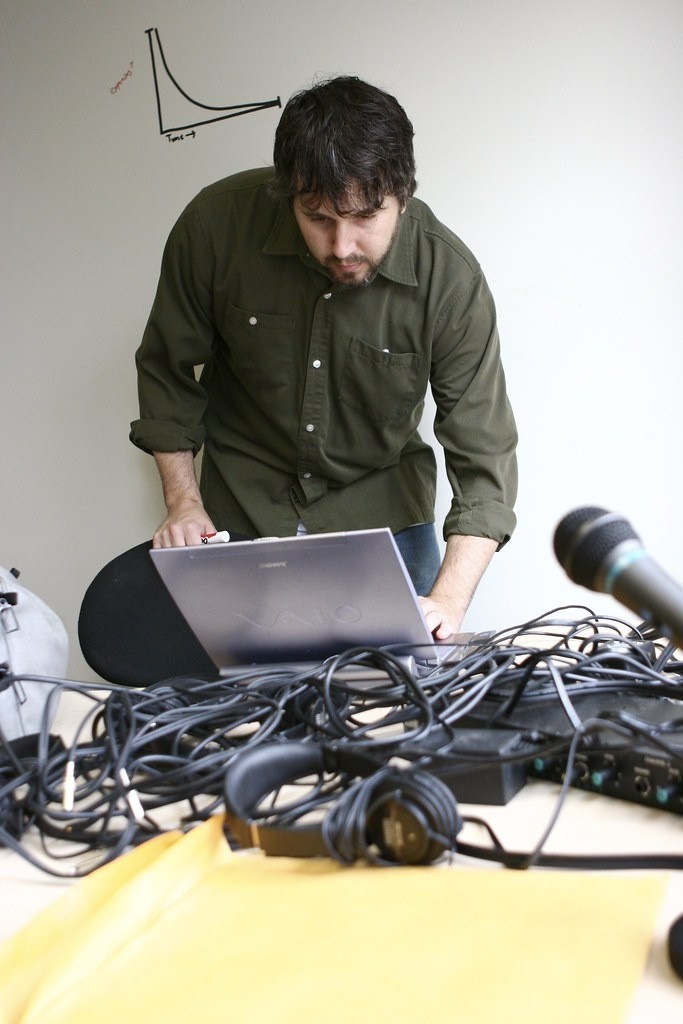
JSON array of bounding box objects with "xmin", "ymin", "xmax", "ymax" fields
[{"xmin": 0, "ymin": 687, "xmax": 683, "ymax": 1024}]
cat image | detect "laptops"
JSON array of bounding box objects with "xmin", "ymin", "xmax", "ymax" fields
[{"xmin": 148, "ymin": 527, "xmax": 478, "ymax": 691}]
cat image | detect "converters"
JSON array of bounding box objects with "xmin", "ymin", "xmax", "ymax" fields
[{"xmin": 360, "ymin": 726, "xmax": 528, "ymax": 806}]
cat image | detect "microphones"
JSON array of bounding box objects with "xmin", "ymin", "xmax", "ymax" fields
[{"xmin": 552, "ymin": 506, "xmax": 683, "ymax": 653}]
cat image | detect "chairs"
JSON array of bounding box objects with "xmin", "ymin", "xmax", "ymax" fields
[{"xmin": 77, "ymin": 531, "xmax": 257, "ymax": 687}]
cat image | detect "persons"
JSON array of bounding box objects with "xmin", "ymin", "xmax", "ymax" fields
[{"xmin": 128, "ymin": 74, "xmax": 518, "ymax": 640}]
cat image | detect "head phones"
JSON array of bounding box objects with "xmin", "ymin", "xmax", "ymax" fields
[{"xmin": 223, "ymin": 742, "xmax": 460, "ymax": 865}]
